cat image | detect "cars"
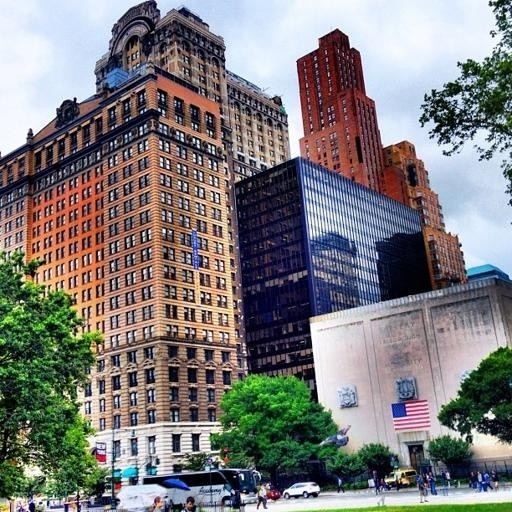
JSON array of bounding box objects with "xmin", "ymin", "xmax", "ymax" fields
[{"xmin": 266, "ymin": 486, "xmax": 281, "ymax": 501}]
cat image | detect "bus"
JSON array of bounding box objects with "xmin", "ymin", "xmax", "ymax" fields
[{"xmin": 136, "ymin": 468, "xmax": 262, "ymax": 507}]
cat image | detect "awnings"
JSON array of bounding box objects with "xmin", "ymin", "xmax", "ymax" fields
[{"xmin": 122, "ymin": 468, "xmax": 137, "ymax": 479}]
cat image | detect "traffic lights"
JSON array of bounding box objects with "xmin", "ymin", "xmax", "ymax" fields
[{"xmin": 223, "ymin": 448, "xmax": 228, "ymax": 453}]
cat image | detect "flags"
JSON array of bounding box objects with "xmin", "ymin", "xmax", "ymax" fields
[{"xmin": 391, "ymin": 399, "xmax": 432, "ymax": 434}]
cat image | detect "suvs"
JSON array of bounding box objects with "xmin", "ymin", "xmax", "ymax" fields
[
  {"xmin": 383, "ymin": 469, "xmax": 419, "ymax": 488},
  {"xmin": 282, "ymin": 481, "xmax": 321, "ymax": 498}
]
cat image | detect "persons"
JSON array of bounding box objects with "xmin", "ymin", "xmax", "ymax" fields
[
  {"xmin": 373, "ymin": 470, "xmax": 391, "ymax": 495},
  {"xmin": 152, "ymin": 496, "xmax": 163, "ymax": 510},
  {"xmin": 416, "ymin": 471, "xmax": 451, "ymax": 503},
  {"xmin": 186, "ymin": 497, "xmax": 195, "ymax": 511},
  {"xmin": 30, "ymin": 500, "xmax": 35, "ymax": 511},
  {"xmin": 257, "ymin": 483, "xmax": 268, "ymax": 509},
  {"xmin": 232, "ymin": 492, "xmax": 246, "ymax": 509},
  {"xmin": 469, "ymin": 469, "xmax": 499, "ymax": 492},
  {"xmin": 337, "ymin": 476, "xmax": 344, "ymax": 493}
]
